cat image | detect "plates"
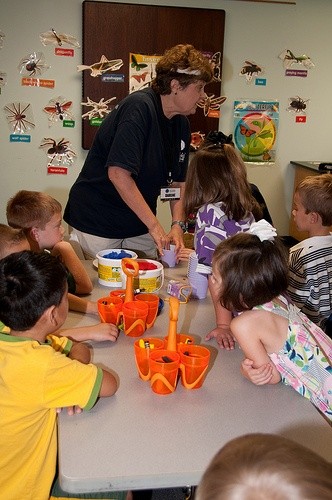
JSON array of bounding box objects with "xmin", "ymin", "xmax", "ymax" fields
[{"xmin": 93, "ymin": 259, "xmax": 98, "ymax": 267}]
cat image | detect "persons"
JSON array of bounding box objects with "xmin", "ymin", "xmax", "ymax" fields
[
  {"xmin": 62, "ymin": 43, "xmax": 215, "ymax": 264},
  {"xmin": 178, "ymin": 129, "xmax": 275, "ymax": 350},
  {"xmin": 212, "ymin": 233, "xmax": 332, "ymax": 422},
  {"xmin": 195, "ymin": 433, "xmax": 332, "ymax": 500},
  {"xmin": 0, "ymin": 190, "xmax": 132, "ymax": 500},
  {"xmin": 286, "ymin": 173, "xmax": 332, "ymax": 333}
]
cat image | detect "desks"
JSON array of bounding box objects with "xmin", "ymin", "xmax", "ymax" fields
[
  {"xmin": 58, "ymin": 258, "xmax": 332, "ymax": 494},
  {"xmin": 289, "ymin": 160, "xmax": 332, "ymax": 242}
]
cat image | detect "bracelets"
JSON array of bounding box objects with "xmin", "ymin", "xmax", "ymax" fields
[{"xmin": 171, "ymin": 221, "xmax": 186, "ymax": 232}]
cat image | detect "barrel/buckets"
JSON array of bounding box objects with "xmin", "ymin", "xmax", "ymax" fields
[
  {"xmin": 122, "ymin": 259, "xmax": 164, "ymax": 294},
  {"xmin": 96, "ymin": 249, "xmax": 138, "ymax": 288}
]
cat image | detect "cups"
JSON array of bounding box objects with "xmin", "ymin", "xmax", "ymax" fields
[
  {"xmin": 122, "ymin": 301, "xmax": 149, "ymax": 337},
  {"xmin": 135, "ymin": 294, "xmax": 159, "ymax": 328},
  {"xmin": 179, "ymin": 345, "xmax": 210, "ymax": 388},
  {"xmin": 164, "ymin": 334, "xmax": 194, "ymax": 353},
  {"xmin": 161, "ymin": 245, "xmax": 176, "ymax": 267},
  {"xmin": 134, "ymin": 338, "xmax": 164, "ymax": 381},
  {"xmin": 97, "ymin": 296, "xmax": 123, "ymax": 326},
  {"xmin": 148, "ymin": 350, "xmax": 180, "ymax": 394},
  {"xmin": 109, "ymin": 290, "xmax": 126, "ymax": 303},
  {"xmin": 187, "ymin": 252, "xmax": 208, "ymax": 300}
]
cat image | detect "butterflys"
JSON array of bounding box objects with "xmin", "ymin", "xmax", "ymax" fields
[
  {"xmin": 205, "ymin": 51, "xmax": 221, "ymax": 82},
  {"xmin": 43, "ymin": 96, "xmax": 74, "ymax": 127},
  {"xmin": 195, "ymin": 92, "xmax": 227, "ymax": 117}
]
